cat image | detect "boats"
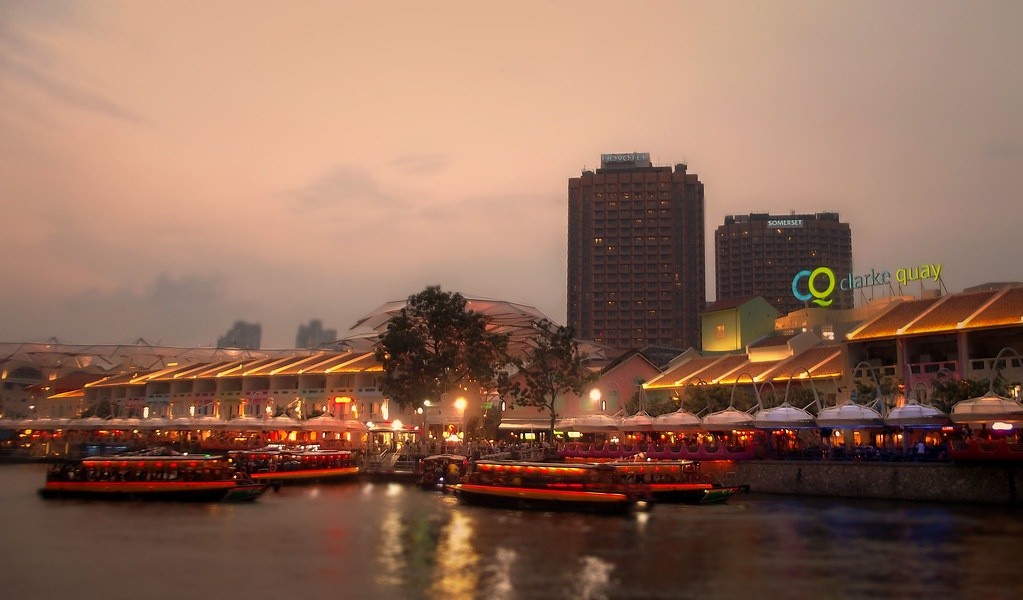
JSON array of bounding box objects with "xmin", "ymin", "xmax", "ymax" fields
[
  {"xmin": 36, "ymin": 442, "xmax": 362, "ymax": 506},
  {"xmin": 417, "ymin": 454, "xmax": 738, "ymax": 517}
]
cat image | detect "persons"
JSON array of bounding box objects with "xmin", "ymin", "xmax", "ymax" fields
[{"xmin": 0, "ymin": 433, "xmax": 1023, "ymax": 482}]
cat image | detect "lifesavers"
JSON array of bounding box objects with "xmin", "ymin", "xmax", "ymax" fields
[
  {"xmin": 269, "ymin": 464, "xmax": 277, "ymax": 472},
  {"xmin": 425, "ymin": 472, "xmax": 434, "ymax": 482}
]
